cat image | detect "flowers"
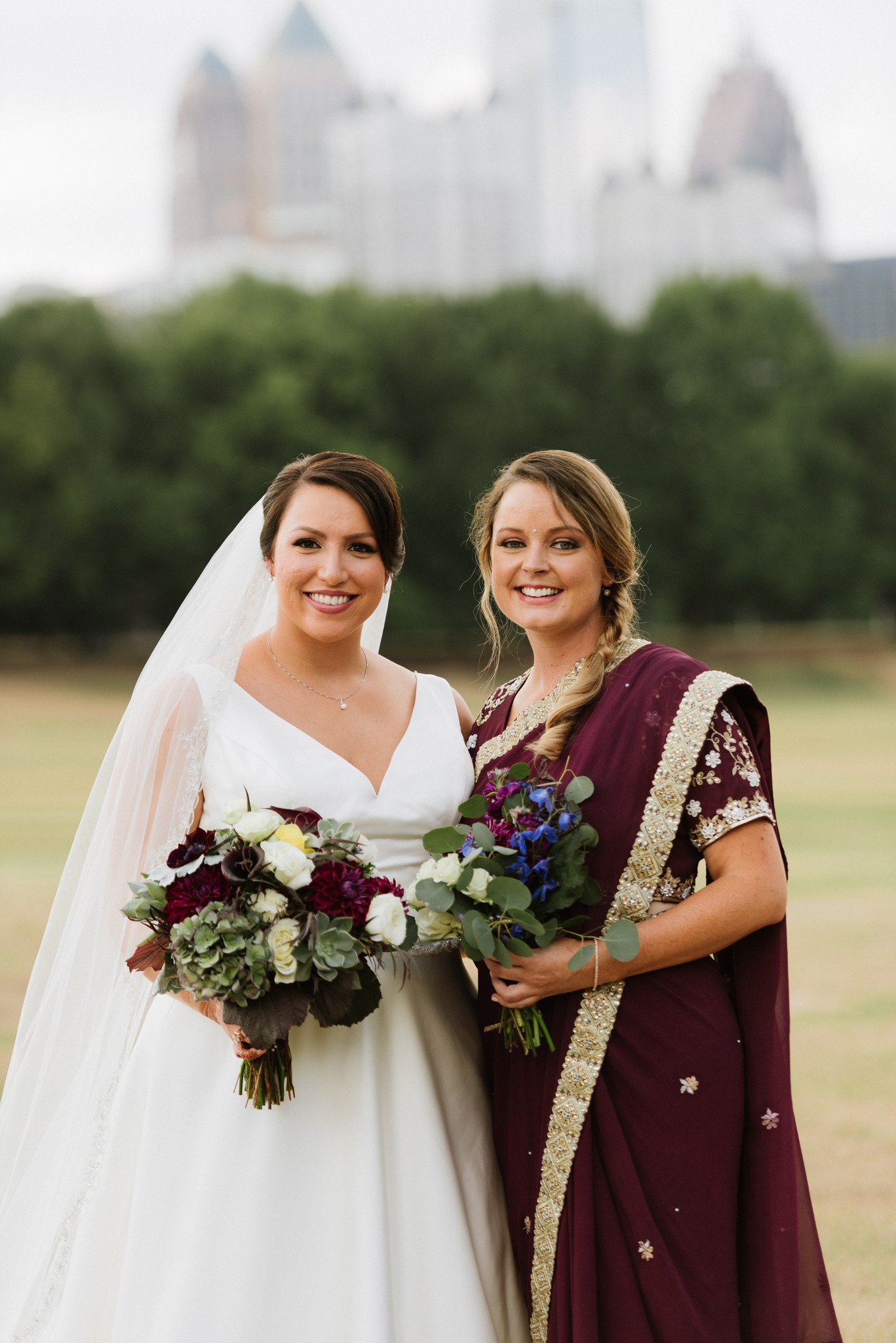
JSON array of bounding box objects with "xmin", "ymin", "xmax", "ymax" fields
[
  {"xmin": 126, "ymin": 799, "xmax": 415, "ymax": 1110},
  {"xmin": 413, "ymin": 760, "xmax": 599, "ymax": 1057}
]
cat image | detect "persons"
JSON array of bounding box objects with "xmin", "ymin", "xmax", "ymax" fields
[
  {"xmin": 457, "ymin": 445, "xmax": 848, "ymax": 1343},
  {"xmin": 0, "ymin": 450, "xmax": 537, "ymax": 1343}
]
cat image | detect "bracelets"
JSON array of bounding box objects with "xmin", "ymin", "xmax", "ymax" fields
[{"xmin": 593, "ymin": 938, "xmax": 599, "ymax": 995}]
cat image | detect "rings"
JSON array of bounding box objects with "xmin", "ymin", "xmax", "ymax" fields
[{"xmin": 232, "ymin": 1027, "xmax": 246, "ymax": 1043}]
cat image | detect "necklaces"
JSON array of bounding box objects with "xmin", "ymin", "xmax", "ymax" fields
[{"xmin": 267, "ymin": 627, "xmax": 370, "ymax": 710}]
